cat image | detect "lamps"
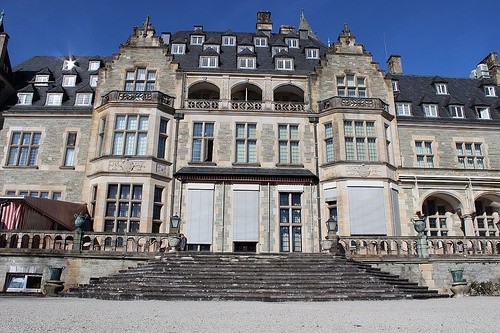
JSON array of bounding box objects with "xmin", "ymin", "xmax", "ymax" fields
[
  {"xmin": 326, "ymin": 216, "xmax": 337, "ymax": 231},
  {"xmin": 170, "ymin": 212, "xmax": 180, "ymax": 228}
]
